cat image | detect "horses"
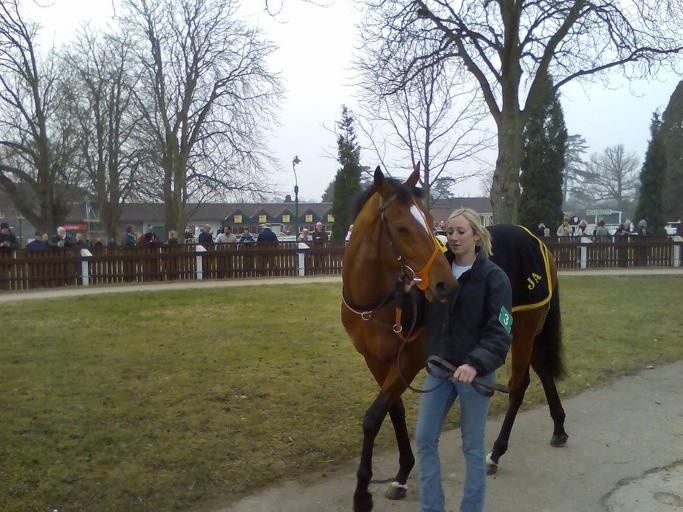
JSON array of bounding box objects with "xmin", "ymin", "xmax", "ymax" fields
[{"xmin": 340, "ymin": 160, "xmax": 572, "ymax": 512}]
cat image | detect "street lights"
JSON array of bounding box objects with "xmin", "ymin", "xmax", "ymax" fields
[
  {"xmin": 17, "ymin": 213, "xmax": 25, "ymax": 248},
  {"xmin": 292, "ymin": 155, "xmax": 302, "ymax": 241}
]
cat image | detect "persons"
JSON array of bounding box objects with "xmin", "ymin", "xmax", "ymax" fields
[
  {"xmin": 199, "ymin": 224, "xmax": 255, "ymax": 267},
  {"xmin": 298, "ymin": 223, "xmax": 328, "ymax": 273},
  {"xmin": 1, "ymin": 223, "xmax": 179, "ymax": 286},
  {"xmin": 345, "ymin": 224, "xmax": 354, "ymax": 240},
  {"xmin": 256, "ymin": 224, "xmax": 279, "ymax": 248},
  {"xmin": 414, "ymin": 206, "xmax": 515, "ymax": 511},
  {"xmin": 534, "ymin": 218, "xmax": 651, "ymax": 268}
]
